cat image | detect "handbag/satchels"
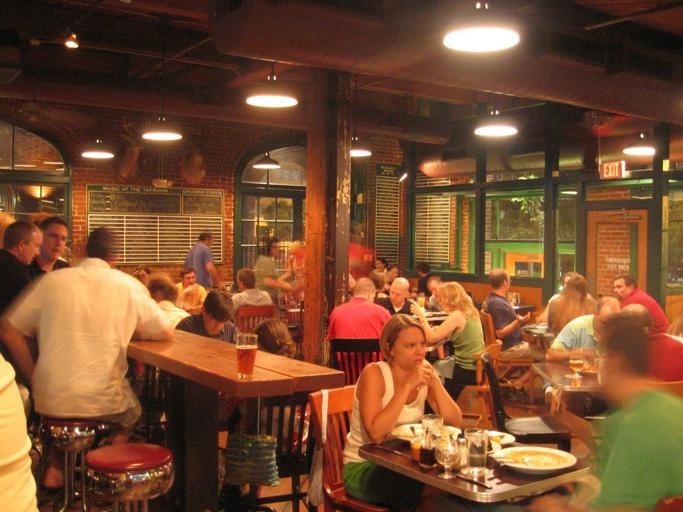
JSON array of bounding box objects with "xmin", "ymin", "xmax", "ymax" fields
[
  {"xmin": 224, "ymin": 397, "xmax": 279, "ymax": 487},
  {"xmin": 306, "ymin": 389, "xmax": 329, "ymax": 507}
]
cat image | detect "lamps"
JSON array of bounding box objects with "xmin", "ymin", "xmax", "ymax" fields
[
  {"xmin": 351, "ymin": 73, "xmax": 373, "ymax": 158},
  {"xmin": 394, "ymin": 167, "xmax": 408, "ymax": 182},
  {"xmin": 442, "ymin": 2, "xmax": 521, "ymax": 53},
  {"xmin": 474, "ymin": 95, "xmax": 518, "ymax": 138},
  {"xmin": 244, "ymin": 62, "xmax": 298, "ymax": 108},
  {"xmin": 142, "ymin": 21, "xmax": 183, "ymax": 141},
  {"xmin": 81, "ymin": 110, "xmax": 116, "ymax": 160},
  {"xmin": 622, "ymin": 130, "xmax": 655, "ymax": 158},
  {"xmin": 252, "ymin": 152, "xmax": 280, "ymax": 169}
]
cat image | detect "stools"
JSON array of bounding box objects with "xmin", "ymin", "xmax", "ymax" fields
[
  {"xmin": 38, "ymin": 418, "xmax": 108, "ymax": 512},
  {"xmin": 85, "ymin": 444, "xmax": 174, "ymax": 512}
]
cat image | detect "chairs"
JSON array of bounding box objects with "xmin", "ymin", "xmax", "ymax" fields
[
  {"xmin": 228, "ymin": 393, "xmax": 308, "ymax": 511},
  {"xmin": 328, "ymin": 338, "xmax": 387, "ymax": 383},
  {"xmin": 235, "ymin": 304, "xmax": 276, "ymax": 332},
  {"xmin": 480, "ymin": 309, "xmax": 535, "ymax": 406},
  {"xmin": 480, "ymin": 351, "xmax": 573, "ymax": 453},
  {"xmin": 283, "ymin": 301, "xmax": 304, "ymax": 358},
  {"xmin": 308, "ymin": 385, "xmax": 397, "ymax": 512}
]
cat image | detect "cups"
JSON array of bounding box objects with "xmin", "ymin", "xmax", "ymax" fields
[
  {"xmin": 236, "ymin": 333, "xmax": 257, "ymax": 380},
  {"xmin": 569, "ymin": 353, "xmax": 585, "ymax": 378},
  {"xmin": 422, "ymin": 414, "xmax": 443, "ymax": 439},
  {"xmin": 418, "ymin": 297, "xmax": 425, "ymax": 311},
  {"xmin": 464, "ymin": 429, "xmax": 488, "ymax": 475},
  {"xmin": 436, "ymin": 439, "xmax": 460, "ymax": 480}
]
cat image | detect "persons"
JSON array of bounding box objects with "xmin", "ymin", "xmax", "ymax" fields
[
  {"xmin": 0, "ymin": 212, "xmax": 16, "ymax": 249},
  {"xmin": 232, "ymin": 269, "xmax": 273, "ymax": 345},
  {"xmin": 488, "ymin": 313, "xmax": 683, "ymax": 512},
  {"xmin": 1, "ymin": 354, "xmax": 38, "ymax": 512},
  {"xmin": 185, "ymin": 230, "xmax": 225, "ymax": 290},
  {"xmin": 668, "ymin": 310, "xmax": 683, "ymax": 340},
  {"xmin": 223, "ymin": 318, "xmax": 309, "ymax": 498},
  {"xmin": 176, "ymin": 288, "xmax": 240, "ymax": 347},
  {"xmin": 342, "ymin": 314, "xmax": 461, "ymax": 512},
  {"xmin": 369, "ymin": 275, "xmax": 388, "ymax": 298},
  {"xmin": 373, "ymin": 276, "xmax": 415, "ymax": 316},
  {"xmin": 482, "ymin": 270, "xmax": 546, "ymax": 400},
  {"xmin": 613, "ymin": 274, "xmax": 669, "ymax": 333},
  {"xmin": 482, "ymin": 269, "xmax": 544, "ymax": 363},
  {"xmin": 384, "ymin": 263, "xmax": 401, "ymax": 286},
  {"xmin": 254, "ymin": 237, "xmax": 293, "ymax": 303},
  {"xmin": 348, "ymin": 259, "xmax": 369, "ymax": 292},
  {"xmin": 426, "ymin": 276, "xmax": 443, "ymax": 310},
  {"xmin": 408, "ymin": 282, "xmax": 482, "ymax": 397},
  {"xmin": 0, "ymin": 221, "xmax": 42, "ymax": 382},
  {"xmin": 147, "ymin": 272, "xmax": 190, "ymax": 329},
  {"xmin": 279, "ymin": 242, "xmax": 306, "ymax": 303},
  {"xmin": 0, "ymin": 227, "xmax": 172, "ymax": 492},
  {"xmin": 132, "ymin": 271, "xmax": 147, "ymax": 284},
  {"xmin": 546, "ymin": 296, "xmax": 621, "ymax": 414},
  {"xmin": 536, "ymin": 271, "xmax": 592, "ymax": 324},
  {"xmin": 410, "ymin": 262, "xmax": 433, "ymax": 299},
  {"xmin": 176, "ymin": 268, "xmax": 207, "ymax": 315},
  {"xmin": 326, "ymin": 277, "xmax": 391, "ymax": 384},
  {"xmin": 373, "ymin": 257, "xmax": 389, "ymax": 276},
  {"xmin": 500, "ymin": 274, "xmax": 597, "ymax": 394},
  {"xmin": 30, "ymin": 217, "xmax": 72, "ymax": 282},
  {"xmin": 135, "ymin": 264, "xmax": 151, "ymax": 275}
]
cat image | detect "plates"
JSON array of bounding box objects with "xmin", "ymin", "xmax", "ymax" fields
[
  {"xmin": 488, "ymin": 441, "xmax": 501, "ymax": 454},
  {"xmin": 493, "ymin": 446, "xmax": 577, "ymax": 475},
  {"xmin": 490, "ymin": 431, "xmax": 515, "ymax": 444},
  {"xmin": 575, "ymin": 366, "xmax": 598, "ymax": 374},
  {"xmin": 392, "ymin": 423, "xmax": 462, "ymax": 442}
]
